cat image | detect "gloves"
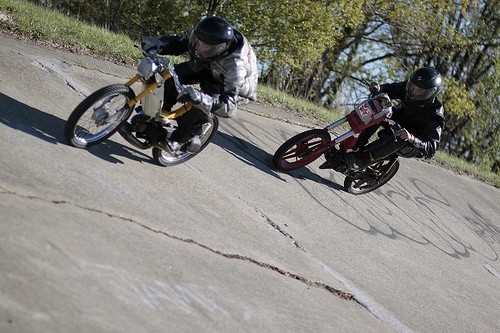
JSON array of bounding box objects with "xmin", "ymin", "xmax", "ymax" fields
[
  {"xmin": 142, "ymin": 39, "xmax": 164, "ymax": 58},
  {"xmin": 177, "ymin": 84, "xmax": 202, "ymax": 105}
]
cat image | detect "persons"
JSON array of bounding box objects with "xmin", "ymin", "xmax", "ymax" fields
[
  {"xmin": 132, "ymin": 16, "xmax": 258, "ymax": 142},
  {"xmin": 353, "ymin": 68, "xmax": 445, "ymax": 169}
]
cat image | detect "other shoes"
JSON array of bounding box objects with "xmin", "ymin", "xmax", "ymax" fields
[
  {"xmin": 347, "ymin": 157, "xmax": 366, "ymax": 172},
  {"xmin": 161, "ymin": 135, "xmax": 181, "ymax": 155}
]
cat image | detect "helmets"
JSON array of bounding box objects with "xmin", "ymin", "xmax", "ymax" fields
[
  {"xmin": 188, "ymin": 15, "xmax": 234, "ymax": 63},
  {"xmin": 405, "ymin": 68, "xmax": 442, "ymax": 106}
]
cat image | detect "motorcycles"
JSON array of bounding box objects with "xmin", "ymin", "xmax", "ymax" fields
[
  {"xmin": 272, "ymin": 77, "xmax": 408, "ymax": 195},
  {"xmin": 64, "ymin": 45, "xmax": 219, "ymax": 167}
]
